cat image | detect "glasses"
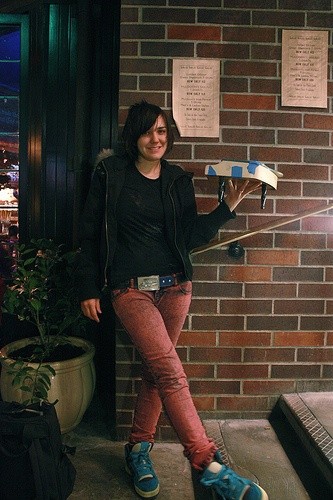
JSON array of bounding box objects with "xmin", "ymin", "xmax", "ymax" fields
[{"xmin": 1, "ymin": 400, "xmax": 46, "ymax": 418}]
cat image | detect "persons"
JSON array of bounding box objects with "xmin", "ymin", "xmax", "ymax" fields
[{"xmin": 78, "ymin": 101, "xmax": 269, "ymax": 500}]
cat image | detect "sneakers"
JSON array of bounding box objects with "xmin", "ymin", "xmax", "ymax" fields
[
  {"xmin": 124, "ymin": 441, "xmax": 160, "ymax": 498},
  {"xmin": 199, "ymin": 451, "xmax": 269, "ymax": 500}
]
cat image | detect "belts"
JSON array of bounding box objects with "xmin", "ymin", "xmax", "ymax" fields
[{"xmin": 117, "ymin": 274, "xmax": 186, "ymax": 291}]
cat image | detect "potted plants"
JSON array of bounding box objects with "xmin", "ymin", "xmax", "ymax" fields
[{"xmin": 0, "ymin": 236, "xmax": 96, "ymax": 435}]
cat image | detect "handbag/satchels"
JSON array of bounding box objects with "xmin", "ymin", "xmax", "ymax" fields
[{"xmin": 0, "ymin": 399, "xmax": 77, "ymax": 500}]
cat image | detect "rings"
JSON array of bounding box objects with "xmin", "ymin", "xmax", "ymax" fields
[{"xmin": 238, "ymin": 190, "xmax": 244, "ymax": 193}]
